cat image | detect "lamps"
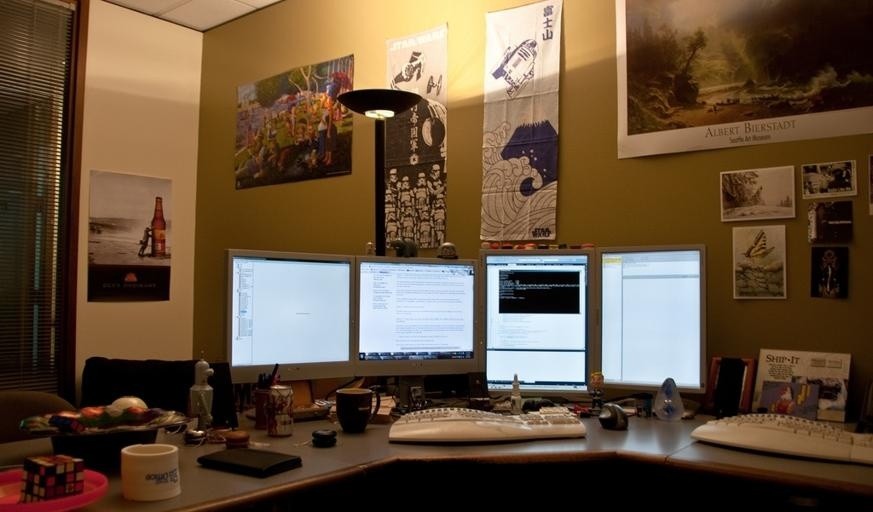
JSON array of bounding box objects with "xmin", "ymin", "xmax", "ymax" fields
[{"xmin": 335, "ymin": 78, "xmax": 423, "ymax": 120}]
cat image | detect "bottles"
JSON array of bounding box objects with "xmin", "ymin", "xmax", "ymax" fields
[
  {"xmin": 510, "ymin": 374, "xmax": 520, "ymax": 413},
  {"xmin": 150, "ymin": 196, "xmax": 165, "ymax": 257}
]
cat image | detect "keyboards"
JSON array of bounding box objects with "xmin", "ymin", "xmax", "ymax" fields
[
  {"xmin": 689, "ymin": 413, "xmax": 873, "ymax": 466},
  {"xmin": 388, "ymin": 405, "xmax": 586, "ymax": 444}
]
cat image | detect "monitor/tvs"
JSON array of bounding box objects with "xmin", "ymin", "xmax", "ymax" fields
[
  {"xmin": 477, "ymin": 248, "xmax": 597, "ymax": 396},
  {"xmin": 354, "ymin": 257, "xmax": 485, "ymax": 401},
  {"xmin": 225, "ymin": 248, "xmax": 353, "ymax": 404},
  {"xmin": 596, "ymin": 244, "xmax": 707, "ymax": 396}
]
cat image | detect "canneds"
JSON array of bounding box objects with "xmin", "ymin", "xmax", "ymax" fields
[{"xmin": 266, "ymin": 384, "xmax": 293, "ymax": 436}]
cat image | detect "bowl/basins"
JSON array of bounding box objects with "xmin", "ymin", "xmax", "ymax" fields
[
  {"xmin": 16, "ymin": 410, "xmax": 190, "ymax": 477},
  {"xmin": 0, "ymin": 465, "xmax": 109, "ymax": 512}
]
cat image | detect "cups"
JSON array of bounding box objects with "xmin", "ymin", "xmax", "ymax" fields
[
  {"xmin": 120, "ymin": 443, "xmax": 181, "ymax": 501},
  {"xmin": 335, "ymin": 388, "xmax": 379, "ymax": 434},
  {"xmin": 253, "ymin": 388, "xmax": 269, "ymax": 430}
]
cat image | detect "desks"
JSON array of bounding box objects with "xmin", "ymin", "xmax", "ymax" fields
[{"xmin": 0, "ymin": 400, "xmax": 872, "ymax": 512}]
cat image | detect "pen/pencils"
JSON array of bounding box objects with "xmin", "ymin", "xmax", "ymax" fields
[{"xmin": 257, "ymin": 363, "xmax": 281, "ymax": 389}]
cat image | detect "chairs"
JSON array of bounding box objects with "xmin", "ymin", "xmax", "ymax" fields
[
  {"xmin": 0, "ymin": 388, "xmax": 78, "ymax": 443},
  {"xmin": 78, "ymin": 355, "xmax": 239, "ymax": 429}
]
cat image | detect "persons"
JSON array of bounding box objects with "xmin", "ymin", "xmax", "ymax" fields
[{"xmin": 136, "ymin": 227, "xmax": 155, "ymax": 257}]
